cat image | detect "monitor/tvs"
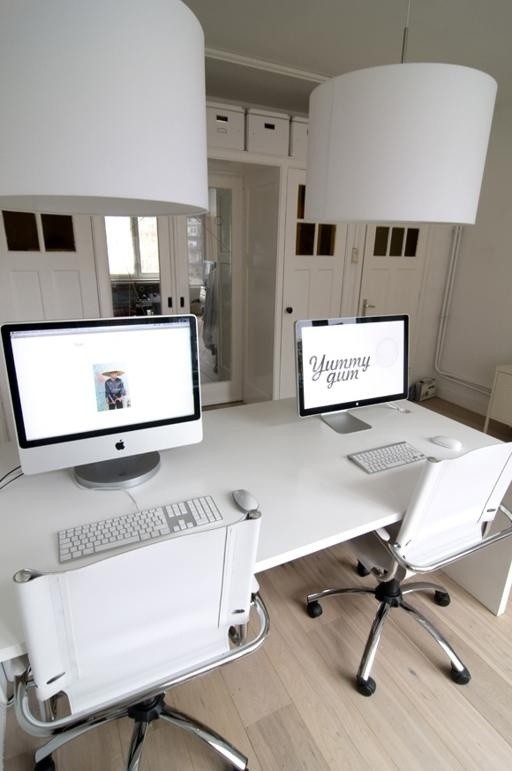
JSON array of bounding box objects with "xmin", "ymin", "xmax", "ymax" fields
[
  {"xmin": 0, "ymin": 312, "xmax": 204, "ymax": 490},
  {"xmin": 293, "ymin": 312, "xmax": 411, "ymax": 434}
]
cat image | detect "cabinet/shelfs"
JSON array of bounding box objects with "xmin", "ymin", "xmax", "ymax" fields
[
  {"xmin": 483, "ymin": 364, "xmax": 512, "ymax": 439},
  {"xmin": 0, "ymin": 208, "xmax": 101, "ymax": 323},
  {"xmin": 243, "ymin": 168, "xmax": 427, "ymax": 403}
]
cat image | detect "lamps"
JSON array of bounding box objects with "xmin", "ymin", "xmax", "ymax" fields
[
  {"xmin": 302, "ymin": 0, "xmax": 498, "ymax": 227},
  {"xmin": 1, "ymin": 1, "xmax": 208, "ymax": 218}
]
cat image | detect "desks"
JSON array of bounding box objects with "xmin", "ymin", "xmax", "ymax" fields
[{"xmin": 0, "ymin": 398, "xmax": 512, "ymax": 718}]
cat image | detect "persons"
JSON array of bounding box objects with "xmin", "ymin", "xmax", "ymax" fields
[{"xmin": 101, "ymin": 368, "xmax": 128, "ymax": 410}]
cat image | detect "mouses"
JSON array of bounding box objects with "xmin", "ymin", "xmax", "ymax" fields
[
  {"xmin": 232, "ymin": 488, "xmax": 260, "ymax": 511},
  {"xmin": 431, "ymin": 435, "xmax": 463, "ymax": 450}
]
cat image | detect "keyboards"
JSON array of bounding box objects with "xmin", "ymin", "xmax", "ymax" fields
[
  {"xmin": 57, "ymin": 493, "xmax": 229, "ymax": 566},
  {"xmin": 346, "ymin": 440, "xmax": 427, "ymax": 475}
]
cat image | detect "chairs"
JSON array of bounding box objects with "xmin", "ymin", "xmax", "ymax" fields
[
  {"xmin": 8, "ymin": 506, "xmax": 274, "ymax": 771},
  {"xmin": 298, "ymin": 438, "xmax": 512, "ymax": 696}
]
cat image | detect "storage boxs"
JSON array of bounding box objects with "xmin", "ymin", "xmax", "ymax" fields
[{"xmin": 206, "ymin": 100, "xmax": 309, "ymax": 162}]
